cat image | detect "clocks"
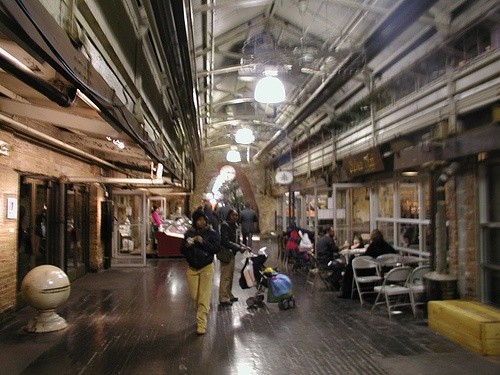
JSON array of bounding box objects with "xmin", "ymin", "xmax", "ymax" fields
[{"xmin": 219, "ymin": 164, "xmax": 237, "ymax": 181}]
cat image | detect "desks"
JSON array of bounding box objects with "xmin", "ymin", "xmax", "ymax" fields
[{"xmin": 333, "ymin": 247, "xmax": 368, "ymax": 297}]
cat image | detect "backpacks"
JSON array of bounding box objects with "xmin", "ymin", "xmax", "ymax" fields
[
  {"xmin": 239, "ymin": 257, "xmax": 253, "ymax": 289},
  {"xmin": 244, "ymin": 258, "xmax": 257, "ymax": 287}
]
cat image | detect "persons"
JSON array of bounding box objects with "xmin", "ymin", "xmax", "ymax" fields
[
  {"xmin": 152, "ymin": 205, "xmax": 158, "ymax": 254},
  {"xmin": 348, "ymin": 234, "xmax": 364, "ymax": 261},
  {"xmin": 282, "ymin": 222, "xmax": 315, "ymax": 273},
  {"xmin": 179, "ymin": 210, "xmax": 222, "ymax": 334},
  {"xmin": 316, "ymin": 225, "xmax": 348, "ymax": 291},
  {"xmin": 336, "ymin": 229, "xmax": 396, "ymax": 299},
  {"xmin": 36, "ymin": 209, "xmax": 48, "ymax": 249},
  {"xmin": 217, "ymin": 209, "xmax": 251, "ymax": 306},
  {"xmin": 197, "ymin": 200, "xmax": 258, "ymax": 252},
  {"xmin": 67, "ymin": 220, "xmax": 73, "ymax": 254},
  {"xmin": 306, "ymin": 199, "xmax": 316, "ymax": 227}
]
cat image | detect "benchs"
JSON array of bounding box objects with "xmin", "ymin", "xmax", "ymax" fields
[{"xmin": 427, "ymin": 299, "xmax": 500, "ymax": 357}]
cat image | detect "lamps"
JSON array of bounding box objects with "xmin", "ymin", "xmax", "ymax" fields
[
  {"xmin": 253, "ymin": 64, "xmax": 286, "ymax": 104},
  {"xmin": 0, "ymin": 139, "xmax": 15, "ymax": 157},
  {"xmin": 234, "ymin": 126, "xmax": 257, "ymax": 144},
  {"xmin": 226, "ymin": 145, "xmax": 242, "ymax": 162},
  {"xmin": 205, "ymin": 174, "xmax": 228, "ymax": 205}
]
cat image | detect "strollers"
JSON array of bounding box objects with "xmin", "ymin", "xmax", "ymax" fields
[{"xmin": 239, "ymin": 247, "xmax": 297, "ymax": 310}]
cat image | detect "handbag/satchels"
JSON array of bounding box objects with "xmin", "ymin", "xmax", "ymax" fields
[{"xmin": 216, "ymin": 244, "xmax": 236, "ymax": 264}]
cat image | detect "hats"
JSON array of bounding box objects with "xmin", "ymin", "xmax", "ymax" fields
[{"xmin": 191, "ymin": 209, "xmax": 209, "ymax": 227}]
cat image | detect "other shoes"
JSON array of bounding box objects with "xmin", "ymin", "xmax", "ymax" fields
[
  {"xmin": 230, "ymin": 297, "xmax": 238, "ymax": 301},
  {"xmin": 197, "ymin": 323, "xmax": 206, "ymax": 334},
  {"xmin": 325, "ymin": 277, "xmax": 339, "ymax": 292},
  {"xmin": 220, "ymin": 301, "xmax": 233, "ymax": 305},
  {"xmin": 353, "ymin": 294, "xmax": 366, "ymax": 300},
  {"xmin": 336, "ymin": 294, "xmax": 352, "ymax": 300}
]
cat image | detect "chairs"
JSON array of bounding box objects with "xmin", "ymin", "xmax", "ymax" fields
[
  {"xmin": 304, "ymin": 249, "xmax": 336, "ymax": 295},
  {"xmin": 351, "ymin": 255, "xmax": 387, "ymax": 306},
  {"xmin": 395, "ymin": 263, "xmax": 434, "ymax": 317},
  {"xmin": 377, "ymin": 253, "xmax": 403, "ymax": 299},
  {"xmin": 369, "ymin": 265, "xmax": 416, "ymax": 321}
]
